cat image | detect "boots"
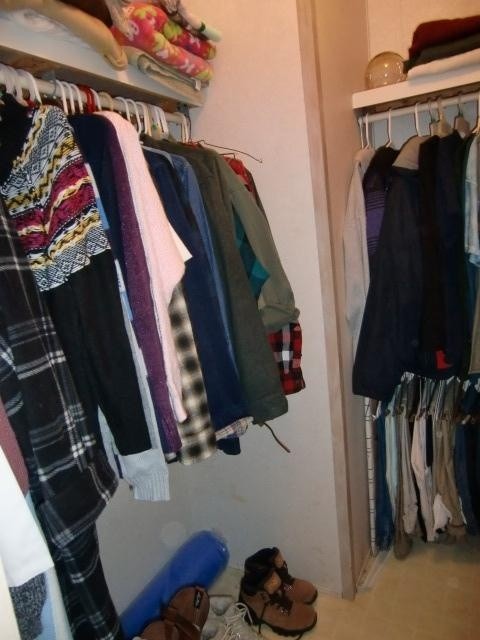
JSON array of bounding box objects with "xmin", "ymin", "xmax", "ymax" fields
[
  {"xmin": 244, "ymin": 546, "xmax": 318, "ymax": 604},
  {"xmin": 238, "ymin": 569, "xmax": 317, "ymax": 639},
  {"xmin": 141, "ymin": 585, "xmax": 211, "ymax": 639},
  {"xmin": 209, "ymin": 595, "xmax": 263, "ymax": 640}
]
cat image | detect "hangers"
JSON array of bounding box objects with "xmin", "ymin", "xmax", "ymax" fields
[
  {"xmin": 0, "ymin": 62, "xmax": 237, "ymax": 163},
  {"xmin": 356, "ymin": 88, "xmax": 480, "ymax": 172},
  {"xmin": 362, "ymin": 370, "xmax": 480, "ymax": 428}
]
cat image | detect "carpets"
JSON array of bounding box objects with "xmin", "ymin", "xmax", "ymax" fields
[{"xmin": 205, "ymin": 567, "xmax": 301, "ymax": 640}]
